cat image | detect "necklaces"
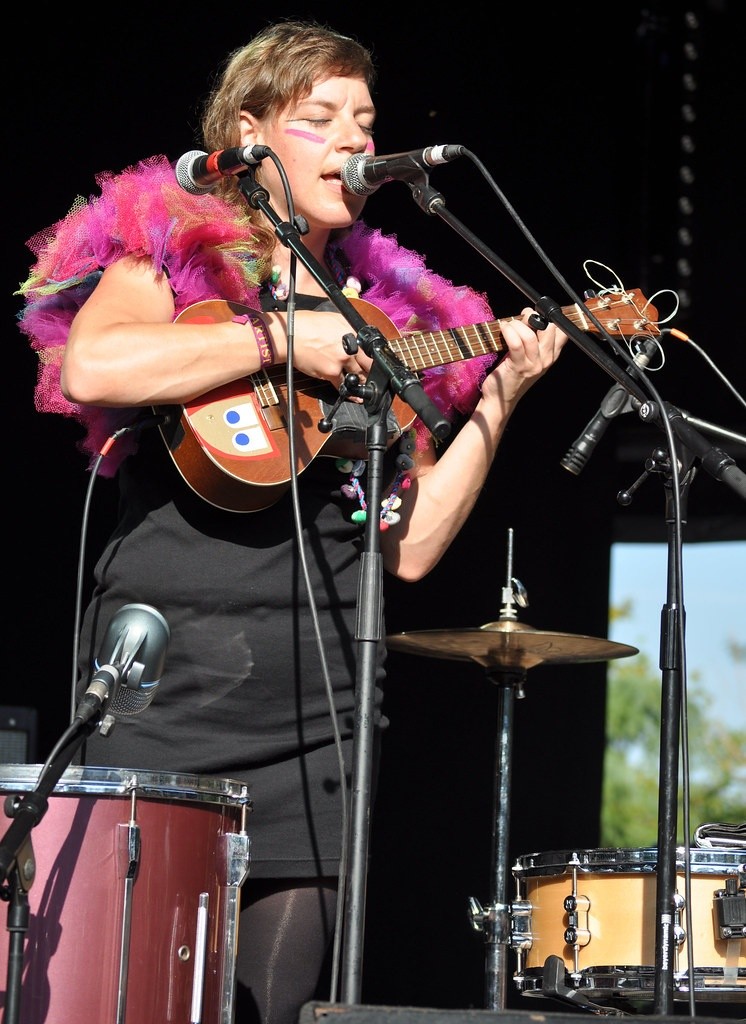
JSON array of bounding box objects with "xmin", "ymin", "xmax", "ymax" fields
[{"xmin": 223, "ymin": 202, "xmax": 424, "ymax": 540}]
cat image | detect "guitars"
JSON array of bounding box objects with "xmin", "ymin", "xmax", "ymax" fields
[{"xmin": 147, "ymin": 257, "xmax": 683, "ymax": 519}]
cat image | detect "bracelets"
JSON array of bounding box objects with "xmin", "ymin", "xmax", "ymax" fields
[{"xmin": 235, "ymin": 306, "xmax": 278, "ymax": 370}]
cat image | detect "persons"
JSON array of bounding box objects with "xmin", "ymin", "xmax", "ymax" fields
[{"xmin": 60, "ymin": 24, "xmax": 567, "ymax": 1020}]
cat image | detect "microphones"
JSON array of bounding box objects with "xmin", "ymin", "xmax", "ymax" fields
[
  {"xmin": 561, "ymin": 332, "xmax": 664, "ymax": 478},
  {"xmin": 174, "ymin": 145, "xmax": 271, "ymax": 195},
  {"xmin": 74, "ymin": 601, "xmax": 170, "ymax": 727},
  {"xmin": 340, "ymin": 144, "xmax": 465, "ymax": 197}
]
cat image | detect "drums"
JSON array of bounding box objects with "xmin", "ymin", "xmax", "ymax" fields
[
  {"xmin": 0, "ymin": 759, "xmax": 259, "ymax": 1024},
  {"xmin": 504, "ymin": 841, "xmax": 746, "ymax": 1010}
]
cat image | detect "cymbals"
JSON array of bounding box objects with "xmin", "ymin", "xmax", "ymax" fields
[{"xmin": 388, "ymin": 619, "xmax": 641, "ymax": 669}]
